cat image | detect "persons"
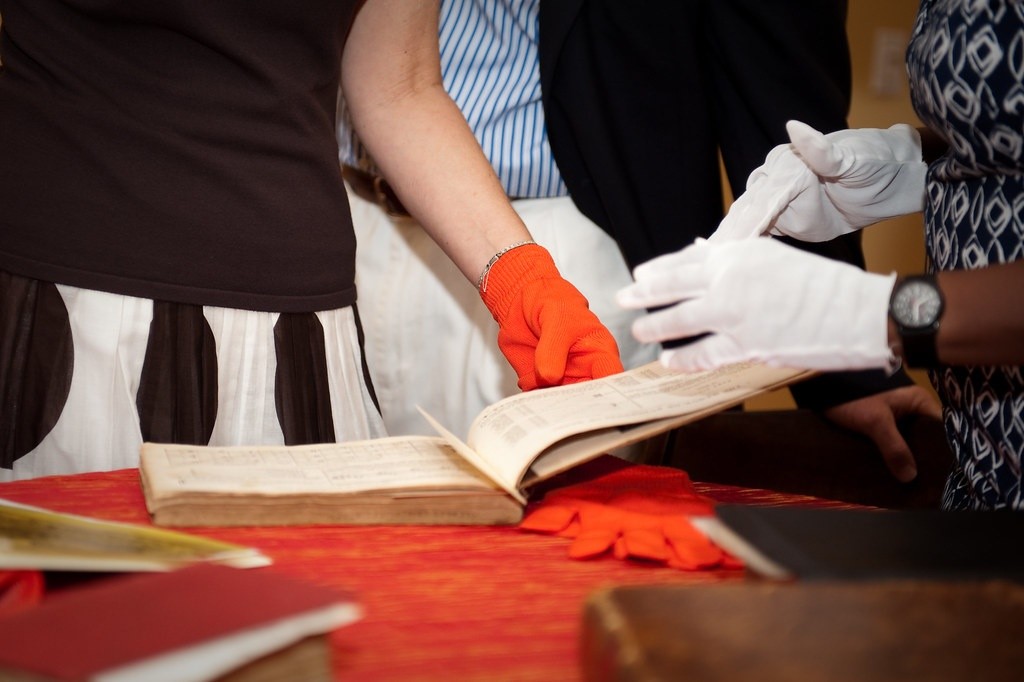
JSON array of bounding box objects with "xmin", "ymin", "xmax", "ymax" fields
[
  {"xmin": 0, "ymin": 0, "xmax": 626, "ymax": 481},
  {"xmin": 334, "ymin": 0, "xmax": 941, "ymax": 481},
  {"xmin": 616, "ymin": 0, "xmax": 1024, "ymax": 511}
]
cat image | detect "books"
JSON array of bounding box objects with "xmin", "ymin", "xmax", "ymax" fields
[
  {"xmin": 139, "ymin": 346, "xmax": 814, "ymax": 529},
  {"xmin": 694, "ymin": 504, "xmax": 1024, "ymax": 582},
  {"xmin": 579, "ymin": 579, "xmax": 1024, "ymax": 682},
  {"xmin": 0, "ymin": 562, "xmax": 362, "ymax": 682}
]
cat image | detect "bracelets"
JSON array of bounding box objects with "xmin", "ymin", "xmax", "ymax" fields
[{"xmin": 478, "ymin": 241, "xmax": 537, "ymax": 293}]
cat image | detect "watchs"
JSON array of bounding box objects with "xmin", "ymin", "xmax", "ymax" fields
[{"xmin": 889, "ymin": 272, "xmax": 945, "ymax": 371}]
